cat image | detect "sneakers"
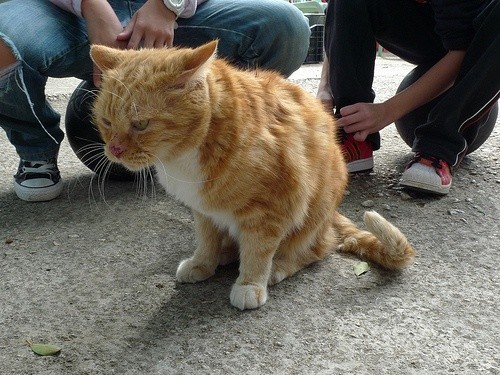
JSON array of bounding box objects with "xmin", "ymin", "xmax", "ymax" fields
[
  {"xmin": 13, "ymin": 158, "xmax": 63, "ymax": 201},
  {"xmin": 339, "ymin": 133, "xmax": 374, "ymax": 173},
  {"xmin": 400, "ymin": 151, "xmax": 452, "ymax": 195}
]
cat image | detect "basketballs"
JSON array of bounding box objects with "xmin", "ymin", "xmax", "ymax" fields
[{"xmin": 65, "ymin": 79, "xmax": 156, "ymax": 183}]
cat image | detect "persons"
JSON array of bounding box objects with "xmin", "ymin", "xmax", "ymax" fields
[
  {"xmin": 0, "ymin": 0, "xmax": 310, "ymax": 202},
  {"xmin": 314, "ymin": 0, "xmax": 500, "ymax": 196}
]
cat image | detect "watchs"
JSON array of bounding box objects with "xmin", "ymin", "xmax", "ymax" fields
[{"xmin": 163, "ymin": 0, "xmax": 186, "ymax": 19}]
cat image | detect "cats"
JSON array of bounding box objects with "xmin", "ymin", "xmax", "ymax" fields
[{"xmin": 67, "ymin": 37, "xmax": 416, "ymax": 309}]
